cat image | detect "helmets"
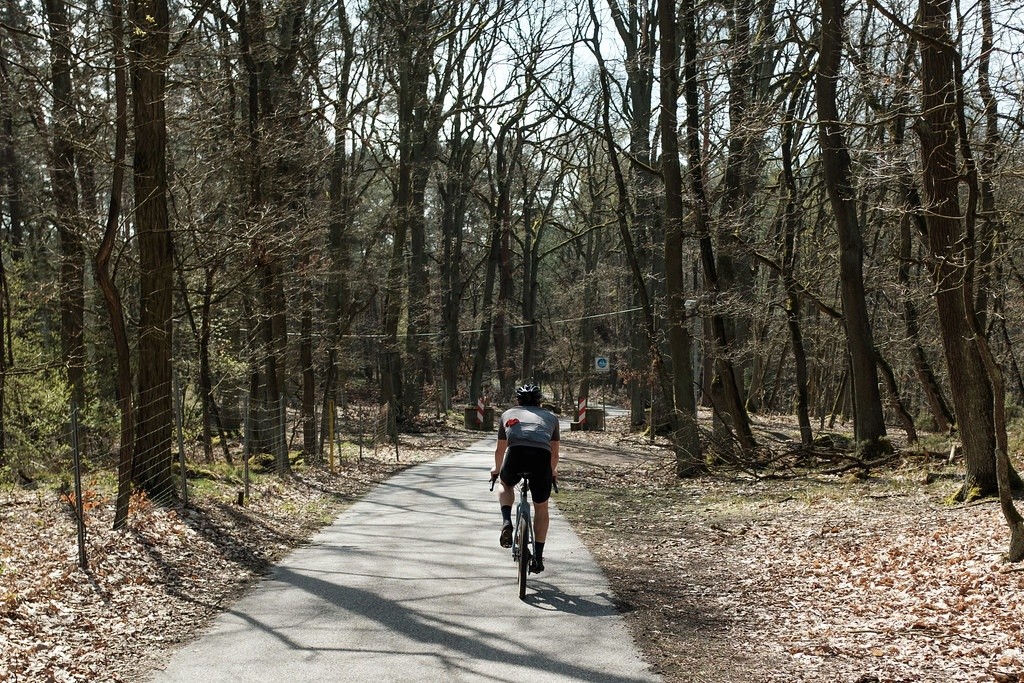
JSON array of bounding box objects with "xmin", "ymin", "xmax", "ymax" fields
[{"xmin": 517, "ymin": 384, "xmax": 542, "ymax": 402}]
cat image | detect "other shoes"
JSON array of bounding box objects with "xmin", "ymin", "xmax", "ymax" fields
[
  {"xmin": 532, "ymin": 562, "xmax": 545, "ymax": 571},
  {"xmin": 500, "ymin": 522, "xmax": 513, "ymax": 548}
]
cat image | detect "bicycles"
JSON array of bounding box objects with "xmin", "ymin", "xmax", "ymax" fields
[{"xmin": 489, "ymin": 472, "xmax": 560, "ymax": 600}]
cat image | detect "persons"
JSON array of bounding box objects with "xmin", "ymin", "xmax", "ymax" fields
[{"xmin": 489, "ymin": 382, "xmax": 561, "ymax": 573}]
